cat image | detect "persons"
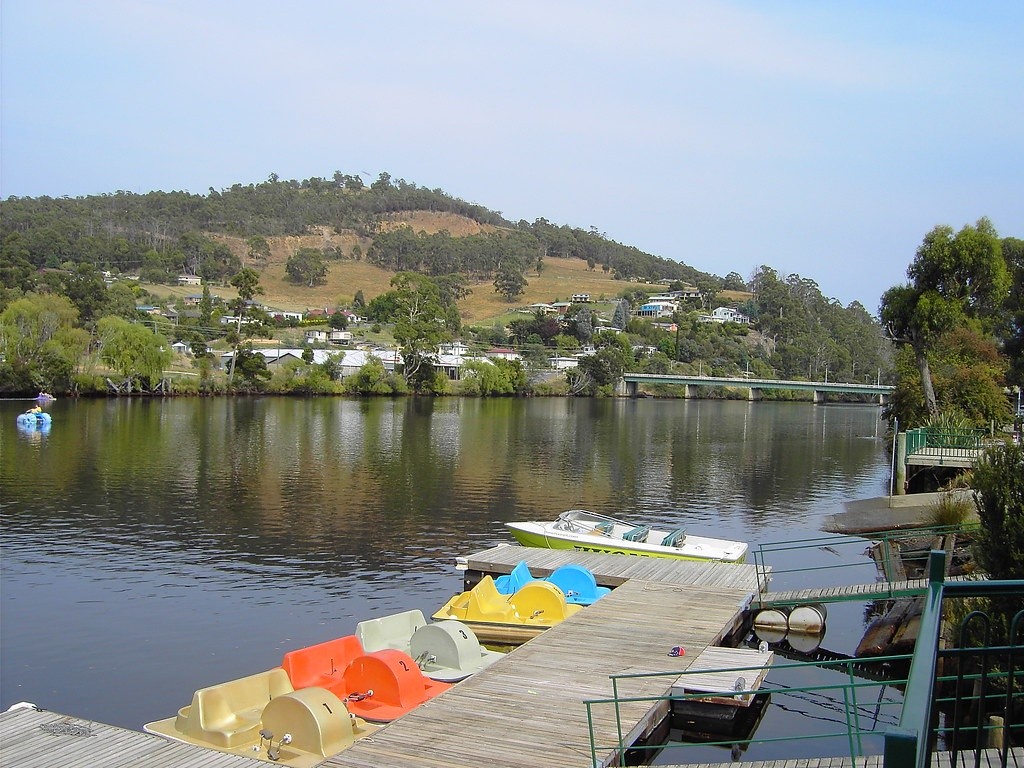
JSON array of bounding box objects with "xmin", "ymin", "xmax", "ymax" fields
[{"xmin": 36, "ymin": 404, "xmax": 42, "ymax": 412}]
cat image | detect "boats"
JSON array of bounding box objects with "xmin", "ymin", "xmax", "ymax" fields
[
  {"xmin": 493, "ymin": 560, "xmax": 612, "ymax": 606},
  {"xmin": 36, "ymin": 395, "xmax": 57, "ymax": 401},
  {"xmin": 142, "ymin": 668, "xmax": 382, "ymax": 768},
  {"xmin": 280, "ymin": 635, "xmax": 455, "ymax": 724},
  {"xmin": 503, "ymin": 509, "xmax": 748, "ymax": 565},
  {"xmin": 354, "ymin": 608, "xmax": 508, "ymax": 683},
  {"xmin": 16, "ymin": 414, "xmax": 52, "ymax": 425},
  {"xmin": 430, "ymin": 575, "xmax": 583, "ymax": 645}
]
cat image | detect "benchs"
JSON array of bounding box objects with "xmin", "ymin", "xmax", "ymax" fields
[
  {"xmin": 357, "ymin": 609, "xmax": 428, "ymax": 652},
  {"xmin": 188, "ymin": 668, "xmax": 295, "ymax": 747},
  {"xmin": 282, "ymin": 635, "xmax": 365, "ymax": 690},
  {"xmin": 471, "ymin": 575, "xmax": 514, "ymax": 622},
  {"xmin": 511, "ymin": 560, "xmax": 543, "ymax": 594},
  {"xmin": 595, "ymin": 521, "xmax": 686, "ymax": 546}
]
